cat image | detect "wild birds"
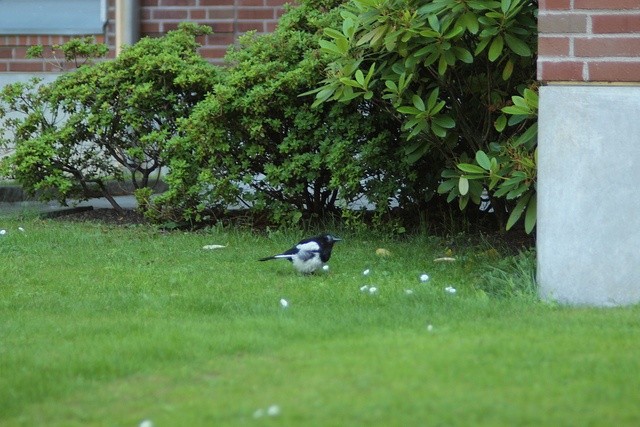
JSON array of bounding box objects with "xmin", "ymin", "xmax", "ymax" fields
[{"xmin": 259, "ymin": 233, "xmax": 342, "ymax": 275}]
